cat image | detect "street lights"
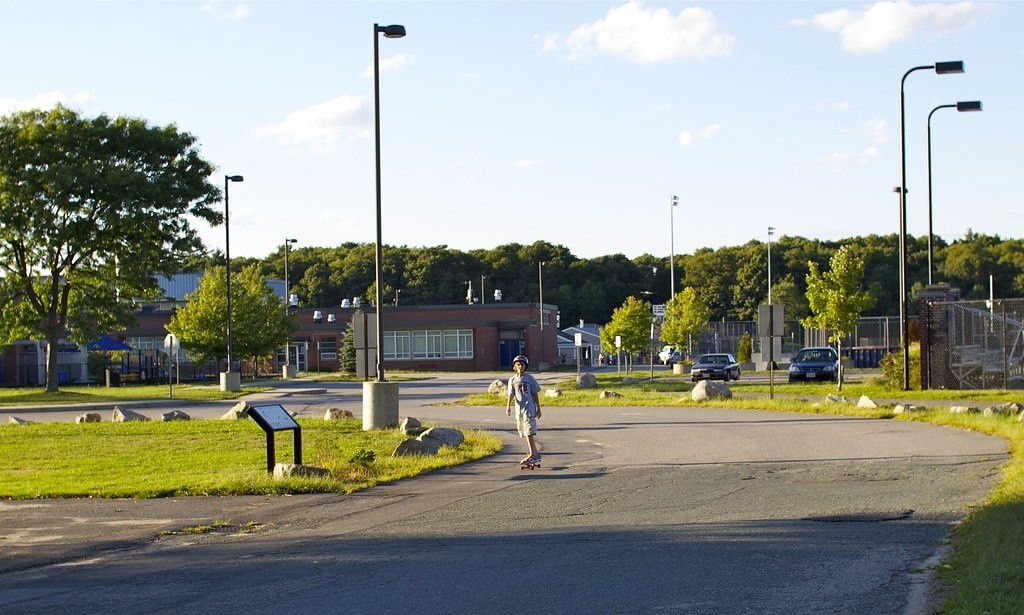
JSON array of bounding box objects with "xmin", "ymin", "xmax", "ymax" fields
[
  {"xmin": 284, "ymin": 238, "xmax": 298, "ymax": 364},
  {"xmin": 224, "ymin": 174, "xmax": 245, "ymax": 371},
  {"xmin": 892, "ymin": 59, "xmax": 968, "ymax": 392},
  {"xmin": 927, "ymin": 99, "xmax": 983, "ymax": 284},
  {"xmin": 372, "ymin": 21, "xmax": 408, "ymax": 380},
  {"xmin": 767, "ymin": 224, "xmax": 777, "ymax": 304},
  {"xmin": 670, "ymin": 193, "xmax": 678, "ymax": 325}
]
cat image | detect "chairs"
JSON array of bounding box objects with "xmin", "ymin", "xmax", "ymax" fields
[{"xmin": 721, "ymin": 360, "xmax": 727, "ymax": 363}]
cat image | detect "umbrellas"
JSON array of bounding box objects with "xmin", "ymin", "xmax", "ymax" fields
[{"xmin": 77, "ymin": 335, "xmax": 133, "ymax": 350}]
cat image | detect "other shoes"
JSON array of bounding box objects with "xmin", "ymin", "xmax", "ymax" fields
[
  {"xmin": 525, "ymin": 456, "xmax": 539, "ymax": 462},
  {"xmin": 536, "ymin": 453, "xmax": 541, "ymax": 460}
]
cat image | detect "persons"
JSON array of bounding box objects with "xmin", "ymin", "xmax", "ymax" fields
[
  {"xmin": 506, "ymin": 356, "xmax": 541, "ymax": 464},
  {"xmin": 599, "ymin": 353, "xmax": 605, "ymax": 366}
]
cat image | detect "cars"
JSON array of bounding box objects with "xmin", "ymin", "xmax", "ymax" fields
[
  {"xmin": 788, "ymin": 346, "xmax": 844, "ymax": 385},
  {"xmin": 690, "ymin": 353, "xmax": 742, "ymax": 382},
  {"xmin": 670, "ymin": 349, "xmax": 690, "ymax": 369},
  {"xmin": 602, "ymin": 351, "xmax": 633, "ymax": 366}
]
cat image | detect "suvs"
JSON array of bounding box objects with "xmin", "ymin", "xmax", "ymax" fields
[{"xmin": 658, "ymin": 344, "xmax": 687, "ymax": 366}]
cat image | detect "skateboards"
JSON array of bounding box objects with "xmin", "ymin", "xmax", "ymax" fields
[{"xmin": 519, "ymin": 459, "xmax": 541, "ymax": 470}]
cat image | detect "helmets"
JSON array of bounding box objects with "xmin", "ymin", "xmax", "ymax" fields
[{"xmin": 513, "ymin": 356, "xmax": 529, "ymax": 368}]
